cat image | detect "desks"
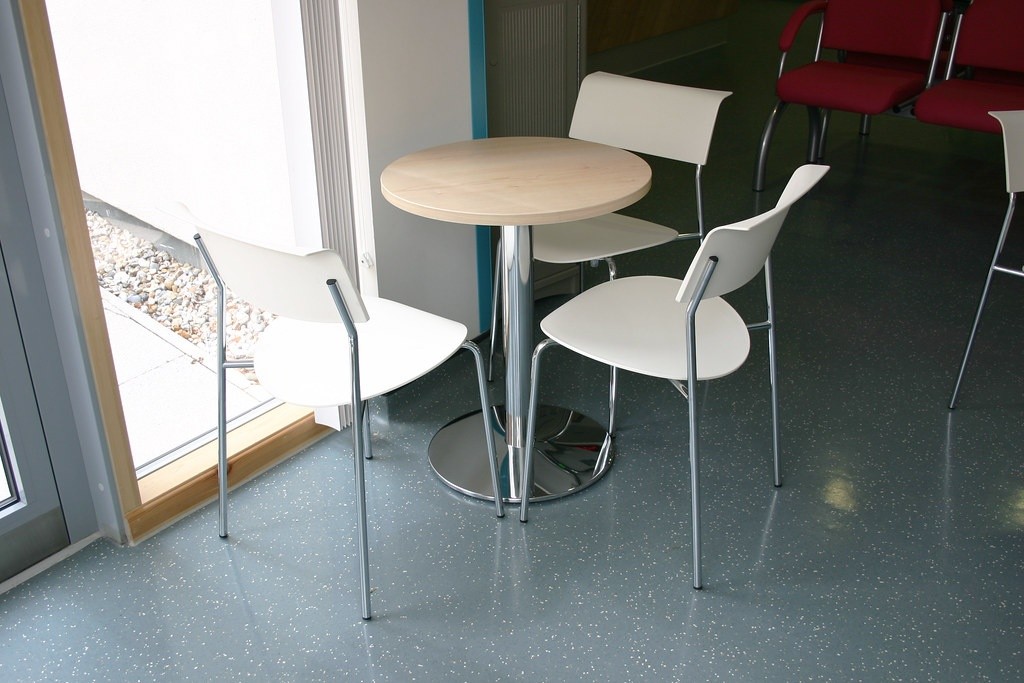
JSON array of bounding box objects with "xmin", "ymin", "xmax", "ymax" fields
[{"xmin": 378, "ymin": 136, "xmax": 654, "ymax": 503}]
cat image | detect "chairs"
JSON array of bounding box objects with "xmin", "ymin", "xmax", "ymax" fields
[
  {"xmin": 915, "ymin": 0, "xmax": 1024, "ymax": 132},
  {"xmin": 520, "ymin": 163, "xmax": 831, "ymax": 591},
  {"xmin": 191, "ymin": 225, "xmax": 506, "ymax": 621},
  {"xmin": 945, "ymin": 108, "xmax": 1024, "ymax": 412},
  {"xmin": 753, "ymin": 1, "xmax": 957, "ymax": 192},
  {"xmin": 486, "ymin": 71, "xmax": 734, "ymax": 413}
]
